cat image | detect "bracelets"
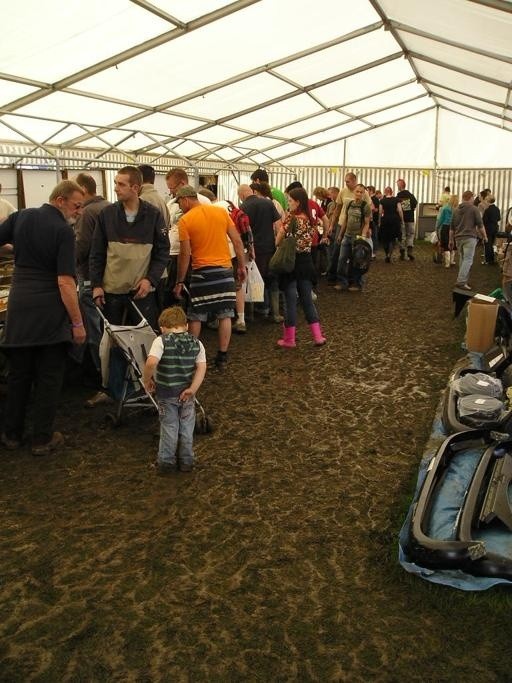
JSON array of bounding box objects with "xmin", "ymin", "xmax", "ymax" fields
[{"xmin": 73, "ymin": 322, "xmax": 84, "ymax": 328}]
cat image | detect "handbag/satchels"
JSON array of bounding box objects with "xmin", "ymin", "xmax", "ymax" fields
[
  {"xmin": 268, "ymin": 216, "xmax": 299, "ymax": 276},
  {"xmin": 433, "ymin": 240, "xmax": 443, "ymax": 265}
]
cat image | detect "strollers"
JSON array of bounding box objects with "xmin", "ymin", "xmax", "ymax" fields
[{"xmin": 90, "ymin": 289, "xmax": 215, "ymax": 453}]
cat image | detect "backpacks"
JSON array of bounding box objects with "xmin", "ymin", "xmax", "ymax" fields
[{"xmin": 225, "ymin": 200, "xmax": 250, "ymax": 234}]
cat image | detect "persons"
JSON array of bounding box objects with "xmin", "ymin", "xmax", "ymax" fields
[
  {"xmin": 74, "ymin": 173, "xmax": 112, "ymax": 372},
  {"xmin": 275, "ymin": 187, "xmax": 327, "ymax": 348},
  {"xmin": 0, "ymin": 182, "xmax": 18, "ymax": 223},
  {"xmin": 250, "ymin": 168, "xmax": 288, "ymax": 210},
  {"xmin": 313, "ymin": 172, "xmax": 383, "ymax": 281},
  {"xmin": 0, "ymin": 179, "xmax": 87, "ymax": 457},
  {"xmin": 165, "ymin": 168, "xmax": 211, "ymax": 308},
  {"xmin": 83, "ymin": 169, "xmax": 171, "ymax": 408},
  {"xmin": 481, "ymin": 195, "xmax": 501, "ymax": 265},
  {"xmin": 448, "ymin": 190, "xmax": 489, "ymax": 293},
  {"xmin": 378, "ymin": 186, "xmax": 403, "ymax": 263},
  {"xmin": 438, "ymin": 187, "xmax": 491, "ymax": 216},
  {"xmin": 435, "ymin": 194, "xmax": 460, "ymax": 268},
  {"xmin": 199, "ymin": 188, "xmax": 255, "ymax": 334},
  {"xmin": 396, "ymin": 177, "xmax": 418, "ymax": 260},
  {"xmin": 333, "ymin": 183, "xmax": 372, "ymax": 293},
  {"xmin": 236, "ymin": 185, "xmax": 284, "ymax": 323},
  {"xmin": 249, "ymin": 183, "xmax": 285, "ymax": 223},
  {"xmin": 176, "ymin": 185, "xmax": 247, "ymax": 366},
  {"xmin": 143, "ymin": 307, "xmax": 207, "ymax": 477},
  {"xmin": 138, "ymin": 164, "xmax": 171, "ymax": 306},
  {"xmin": 284, "ymin": 181, "xmax": 329, "ymax": 266}
]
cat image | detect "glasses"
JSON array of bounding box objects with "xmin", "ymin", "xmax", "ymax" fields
[
  {"xmin": 62, "ymin": 196, "xmax": 85, "ymax": 211},
  {"xmin": 168, "ymin": 182, "xmax": 181, "ymax": 191}
]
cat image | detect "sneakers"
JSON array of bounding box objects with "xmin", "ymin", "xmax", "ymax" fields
[
  {"xmin": 214, "ymin": 358, "xmax": 227, "ymax": 370},
  {"xmin": 85, "ymin": 392, "xmax": 116, "ymax": 408},
  {"xmin": 31, "ymin": 432, "xmax": 65, "ymax": 457},
  {"xmin": 1, "ymin": 432, "xmax": 20, "ymax": 451},
  {"xmin": 156, "ymin": 458, "xmax": 174, "ymax": 476},
  {"xmin": 334, "ymin": 283, "xmax": 343, "ymax": 292},
  {"xmin": 230, "ymin": 322, "xmax": 246, "ymax": 334},
  {"xmin": 456, "ymin": 284, "xmax": 471, "ymax": 291},
  {"xmin": 206, "ymin": 320, "xmax": 219, "ymax": 330},
  {"xmin": 348, "ymin": 284, "xmax": 363, "ymax": 292},
  {"xmin": 180, "ymin": 461, "xmax": 194, "ymax": 473}
]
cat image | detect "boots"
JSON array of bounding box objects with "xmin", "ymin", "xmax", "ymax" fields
[
  {"xmin": 407, "ymin": 246, "xmax": 415, "ymax": 261},
  {"xmin": 451, "ymin": 250, "xmax": 456, "ymax": 267},
  {"xmin": 310, "ymin": 322, "xmax": 327, "ymax": 347},
  {"xmin": 399, "ymin": 250, "xmax": 406, "ymax": 261},
  {"xmin": 444, "ymin": 250, "xmax": 450, "ymax": 269},
  {"xmin": 276, "ymin": 323, "xmax": 296, "ymax": 348},
  {"xmin": 268, "ymin": 293, "xmax": 284, "ymax": 324}
]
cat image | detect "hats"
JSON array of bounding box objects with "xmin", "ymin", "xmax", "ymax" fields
[
  {"xmin": 199, "ymin": 189, "xmax": 217, "ymax": 203},
  {"xmin": 168, "ymin": 185, "xmax": 196, "ymax": 204}
]
